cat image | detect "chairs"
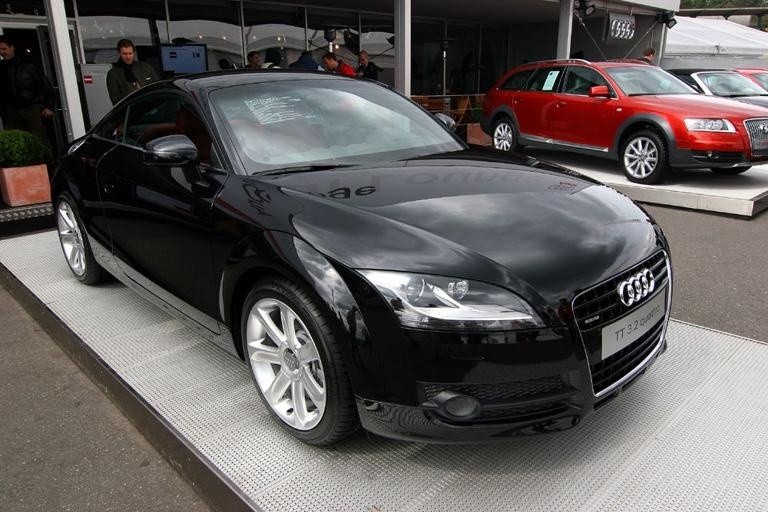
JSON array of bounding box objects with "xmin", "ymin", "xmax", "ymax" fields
[{"xmin": 410, "ymin": 93, "xmax": 488, "ymax": 133}]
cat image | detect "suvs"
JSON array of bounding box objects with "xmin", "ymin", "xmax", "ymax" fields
[{"xmin": 479, "ymin": 57, "xmax": 768, "ymax": 184}]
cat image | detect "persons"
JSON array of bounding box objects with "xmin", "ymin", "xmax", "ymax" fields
[
  {"xmin": 245, "ymin": 48, "xmax": 384, "ymax": 81},
  {"xmin": 637, "ymin": 47, "xmax": 655, "ymax": 65},
  {"xmin": 106, "ymin": 39, "xmax": 160, "ymax": 106},
  {"xmin": 0, "ymin": 35, "xmax": 56, "ymax": 148}
]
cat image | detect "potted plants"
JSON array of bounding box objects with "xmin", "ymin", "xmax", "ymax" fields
[{"xmin": 0, "ymin": 127, "xmax": 56, "ymax": 209}]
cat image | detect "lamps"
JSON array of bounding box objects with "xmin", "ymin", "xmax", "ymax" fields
[
  {"xmin": 654, "ymin": 12, "xmax": 677, "ymax": 29},
  {"xmin": 575, "ymin": 0, "xmax": 596, "ymax": 16}
]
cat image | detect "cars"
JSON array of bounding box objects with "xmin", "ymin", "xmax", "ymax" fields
[
  {"xmin": 664, "ymin": 67, "xmax": 768, "ymax": 108},
  {"xmin": 734, "ymin": 69, "xmax": 768, "ymax": 91},
  {"xmin": 51, "ymin": 66, "xmax": 673, "ymax": 449}
]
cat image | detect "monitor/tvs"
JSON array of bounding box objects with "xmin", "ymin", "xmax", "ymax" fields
[{"xmin": 160, "ymin": 43, "xmax": 208, "ymax": 73}]
